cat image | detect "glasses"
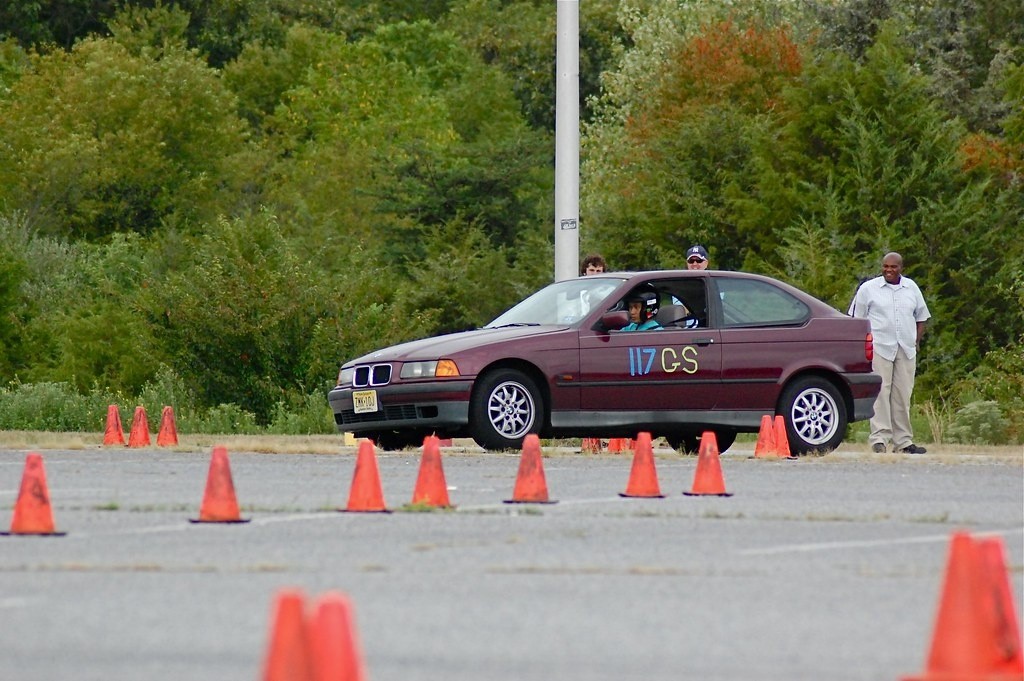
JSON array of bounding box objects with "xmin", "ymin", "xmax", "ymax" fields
[{"xmin": 688, "ymin": 259, "xmax": 703, "ymax": 263}]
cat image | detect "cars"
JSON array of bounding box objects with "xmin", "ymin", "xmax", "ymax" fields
[{"xmin": 326, "ymin": 268, "xmax": 883, "ymax": 458}]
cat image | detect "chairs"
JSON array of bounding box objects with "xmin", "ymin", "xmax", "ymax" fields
[{"xmin": 658, "ymin": 306, "xmax": 686, "ymax": 330}]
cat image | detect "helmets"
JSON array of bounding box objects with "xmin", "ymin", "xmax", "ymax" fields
[{"xmin": 624, "ymin": 283, "xmax": 660, "ymax": 322}]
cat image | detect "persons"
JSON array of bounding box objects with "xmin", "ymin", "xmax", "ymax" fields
[
  {"xmin": 565, "ymin": 254, "xmax": 609, "ymax": 300},
  {"xmin": 617, "ymin": 286, "xmax": 664, "ymax": 332},
  {"xmin": 847, "ymin": 252, "xmax": 931, "ymax": 454},
  {"xmin": 671, "ymin": 245, "xmax": 725, "ymax": 329}
]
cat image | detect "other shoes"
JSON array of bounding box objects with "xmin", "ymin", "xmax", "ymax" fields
[
  {"xmin": 893, "ymin": 444, "xmax": 926, "ymax": 454},
  {"xmin": 873, "ymin": 443, "xmax": 886, "ymax": 453}
]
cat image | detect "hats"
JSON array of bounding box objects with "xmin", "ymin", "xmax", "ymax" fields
[{"xmin": 686, "ymin": 246, "xmax": 708, "ymax": 260}]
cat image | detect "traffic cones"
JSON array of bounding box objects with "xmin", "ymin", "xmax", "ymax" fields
[
  {"xmin": 617, "ymin": 431, "xmax": 665, "ymax": 498},
  {"xmin": 747, "ymin": 415, "xmax": 785, "ymax": 460},
  {"xmin": 682, "ymin": 433, "xmax": 733, "ymax": 497},
  {"xmin": 125, "ymin": 407, "xmax": 151, "ymax": 448},
  {"xmin": 97, "ymin": 405, "xmax": 128, "ymax": 447},
  {"xmin": 501, "ymin": 434, "xmax": 558, "ymax": 504},
  {"xmin": 335, "ymin": 441, "xmax": 393, "ymax": 513},
  {"xmin": 773, "ymin": 416, "xmax": 798, "ymax": 461},
  {"xmin": 310, "ymin": 595, "xmax": 364, "ymax": 681},
  {"xmin": 157, "ymin": 406, "xmax": 179, "ymax": 446},
  {"xmin": 402, "ymin": 436, "xmax": 458, "ymax": 509},
  {"xmin": 898, "ymin": 533, "xmax": 1024, "ymax": 681},
  {"xmin": 187, "ymin": 447, "xmax": 252, "ymax": 524},
  {"xmin": 257, "ymin": 595, "xmax": 314, "ymax": 681},
  {"xmin": 0, "ymin": 454, "xmax": 68, "ymax": 536}
]
